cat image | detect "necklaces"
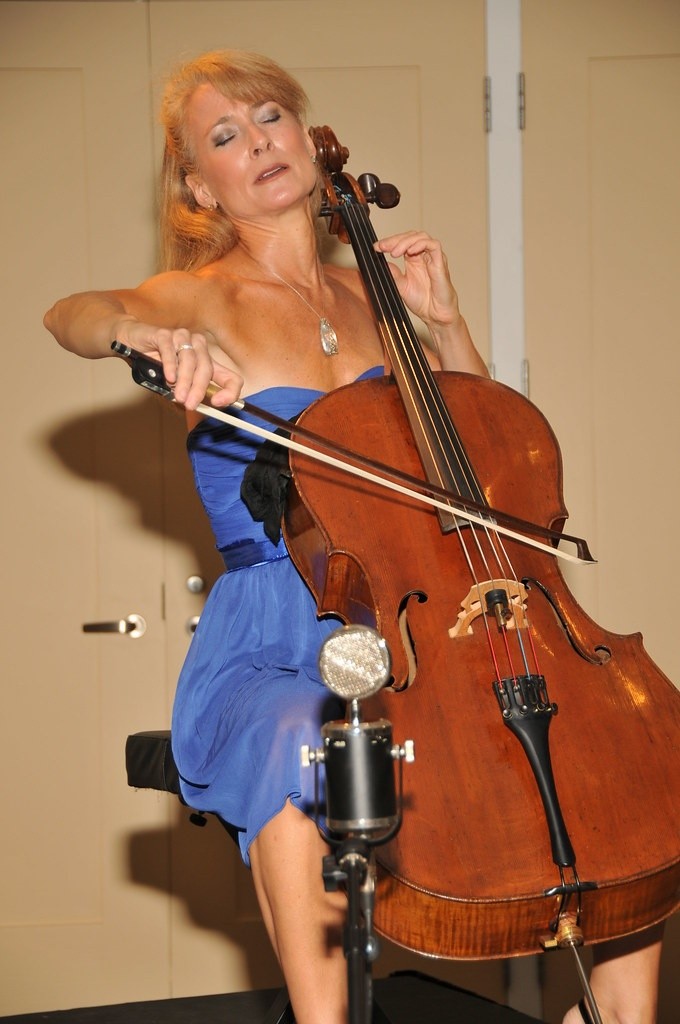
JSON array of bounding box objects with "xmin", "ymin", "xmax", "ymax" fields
[{"xmin": 248, "ymin": 251, "xmax": 337, "ymax": 356}]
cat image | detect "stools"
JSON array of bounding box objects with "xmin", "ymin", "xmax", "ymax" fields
[{"xmin": 126, "ymin": 731, "xmax": 393, "ymax": 1024}]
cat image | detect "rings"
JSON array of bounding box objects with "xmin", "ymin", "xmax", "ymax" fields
[{"xmin": 177, "ymin": 343, "xmax": 194, "ymax": 355}]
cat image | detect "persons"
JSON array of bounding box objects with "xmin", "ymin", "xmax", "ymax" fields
[{"xmin": 39, "ymin": 51, "xmax": 664, "ymax": 1022}]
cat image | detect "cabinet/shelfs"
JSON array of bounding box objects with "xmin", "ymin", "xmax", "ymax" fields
[{"xmin": 0, "ymin": 0, "xmax": 680, "ymax": 1024}]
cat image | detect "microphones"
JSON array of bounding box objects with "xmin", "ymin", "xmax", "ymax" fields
[{"xmin": 316, "ymin": 623, "xmax": 398, "ymax": 831}]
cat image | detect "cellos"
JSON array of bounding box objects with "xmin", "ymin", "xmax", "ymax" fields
[{"xmin": 248, "ymin": 122, "xmax": 680, "ymax": 1023}]
